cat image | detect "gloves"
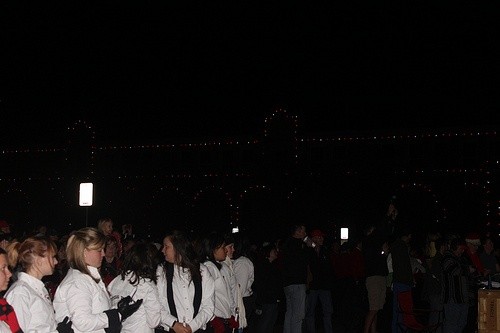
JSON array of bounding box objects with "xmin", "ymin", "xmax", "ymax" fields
[
  {"xmin": 117, "ymin": 295, "xmax": 135, "ymax": 306},
  {"xmin": 56, "ymin": 315, "xmax": 74, "ymax": 332},
  {"xmin": 119, "ymin": 298, "xmax": 143, "ymax": 321}
]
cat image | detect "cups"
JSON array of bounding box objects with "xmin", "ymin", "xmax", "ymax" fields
[
  {"xmin": 410, "ymin": 258, "xmax": 420, "ymax": 272},
  {"xmin": 303, "ymin": 236, "xmax": 316, "ymax": 248}
]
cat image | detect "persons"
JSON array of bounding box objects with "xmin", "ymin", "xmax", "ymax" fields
[
  {"xmin": 200, "ymin": 234, "xmax": 247, "ymax": 333},
  {"xmin": 107, "ymin": 236, "xmax": 162, "ymax": 333},
  {"xmin": 0, "ymin": 219, "xmax": 221, "ymax": 333},
  {"xmin": 220, "ymin": 232, "xmax": 282, "ymax": 333},
  {"xmin": 3, "ymin": 235, "xmax": 74, "ymax": 333},
  {"xmin": 53, "ymin": 227, "xmax": 143, "ymax": 333},
  {"xmin": 305, "ymin": 228, "xmax": 499, "ymax": 333},
  {"xmin": 279, "ymin": 223, "xmax": 320, "ymax": 333},
  {"xmin": 154, "ymin": 228, "xmax": 215, "ymax": 333}
]
